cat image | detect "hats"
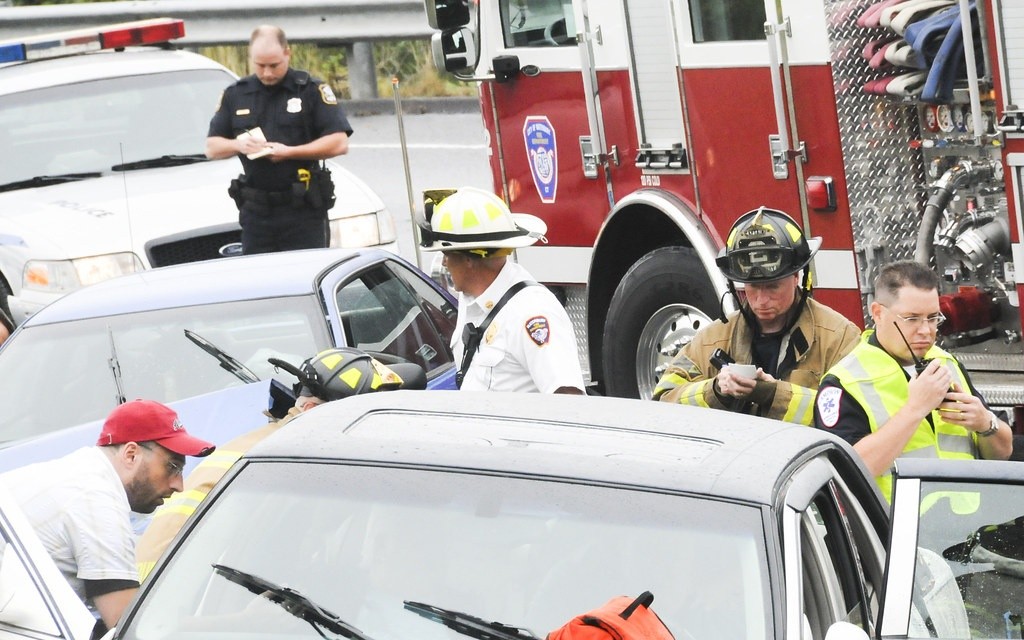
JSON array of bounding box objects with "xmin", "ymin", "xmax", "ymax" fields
[{"xmin": 96, "ymin": 399, "xmax": 216, "ymax": 457}]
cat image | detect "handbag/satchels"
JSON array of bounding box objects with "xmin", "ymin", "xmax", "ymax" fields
[{"xmin": 544, "ymin": 591, "xmax": 675, "ymax": 640}]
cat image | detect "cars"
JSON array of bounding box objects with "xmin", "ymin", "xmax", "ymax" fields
[
  {"xmin": 0, "ymin": 16, "xmax": 403, "ymax": 338},
  {"xmin": 1, "ymin": 249, "xmax": 473, "ymax": 573},
  {"xmin": 1, "ymin": 391, "xmax": 1023, "ymax": 639}
]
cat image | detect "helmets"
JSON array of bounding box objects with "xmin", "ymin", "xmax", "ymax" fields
[
  {"xmin": 715, "ymin": 206, "xmax": 823, "ymax": 283},
  {"xmin": 267, "ymin": 348, "xmax": 405, "ymax": 402},
  {"xmin": 417, "ymin": 187, "xmax": 549, "ymax": 251}
]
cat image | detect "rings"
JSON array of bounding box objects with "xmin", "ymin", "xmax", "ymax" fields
[{"xmin": 960, "ymin": 412, "xmax": 963, "ymax": 420}]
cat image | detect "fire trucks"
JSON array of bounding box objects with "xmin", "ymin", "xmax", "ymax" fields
[{"xmin": 377, "ymin": 0, "xmax": 1024, "ymax": 429}]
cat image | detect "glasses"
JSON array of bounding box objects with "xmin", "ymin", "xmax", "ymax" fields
[
  {"xmin": 442, "ymin": 251, "xmax": 472, "ymax": 255},
  {"xmin": 880, "ymin": 303, "xmax": 946, "ymax": 327}
]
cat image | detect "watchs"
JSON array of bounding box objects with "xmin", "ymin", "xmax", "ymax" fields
[{"xmin": 974, "ymin": 410, "xmax": 999, "ymax": 437}]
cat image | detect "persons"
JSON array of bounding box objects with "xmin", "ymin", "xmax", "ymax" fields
[
  {"xmin": 813, "ymin": 260, "xmax": 1013, "ymax": 604},
  {"xmin": 136, "ymin": 347, "xmax": 404, "ymax": 588},
  {"xmin": 414, "ymin": 187, "xmax": 587, "ymax": 396},
  {"xmin": 204, "ymin": 26, "xmax": 353, "ymax": 255},
  {"xmin": 0, "ymin": 322, "xmax": 9, "ymax": 348},
  {"xmin": 0, "ymin": 399, "xmax": 311, "ymax": 640},
  {"xmin": 651, "ymin": 205, "xmax": 862, "ymax": 429}
]
